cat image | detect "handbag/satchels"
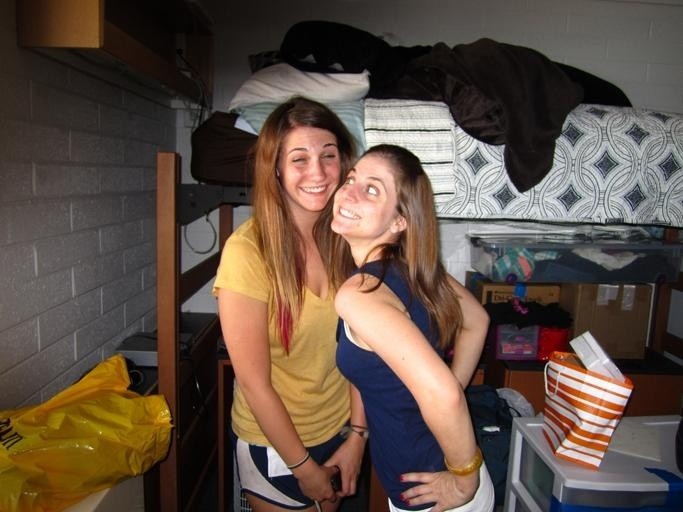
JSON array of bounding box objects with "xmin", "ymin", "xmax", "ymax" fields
[
  {"xmin": 465, "ymin": 383, "xmax": 523, "ymax": 487},
  {"xmin": 542, "ymin": 352, "xmax": 632, "ymax": 469}
]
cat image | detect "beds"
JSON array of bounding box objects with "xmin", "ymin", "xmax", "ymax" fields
[{"xmin": 197, "ymin": 57, "xmax": 683, "ymax": 215}]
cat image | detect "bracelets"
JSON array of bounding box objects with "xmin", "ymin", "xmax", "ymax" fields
[
  {"xmin": 444, "ymin": 446, "xmax": 483, "ymax": 477},
  {"xmin": 287, "ymin": 451, "xmax": 310, "ymax": 470},
  {"xmin": 350, "ymin": 425, "xmax": 369, "ymax": 439}
]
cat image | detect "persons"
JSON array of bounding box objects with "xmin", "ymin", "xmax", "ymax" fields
[
  {"xmin": 212, "ymin": 96, "xmax": 370, "ymax": 512},
  {"xmin": 331, "ymin": 145, "xmax": 497, "ymax": 512}
]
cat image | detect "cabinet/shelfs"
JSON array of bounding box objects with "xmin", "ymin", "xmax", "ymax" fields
[
  {"xmin": 111, "ymin": 309, "xmax": 223, "ymax": 512},
  {"xmin": 15, "ymin": 0, "xmax": 218, "ymax": 111}
]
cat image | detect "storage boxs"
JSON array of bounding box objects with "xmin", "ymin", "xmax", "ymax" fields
[
  {"xmin": 476, "ymin": 236, "xmax": 683, "ymax": 285},
  {"xmin": 467, "ymin": 268, "xmax": 559, "ymax": 312},
  {"xmin": 563, "ymin": 279, "xmax": 650, "ymax": 363}
]
